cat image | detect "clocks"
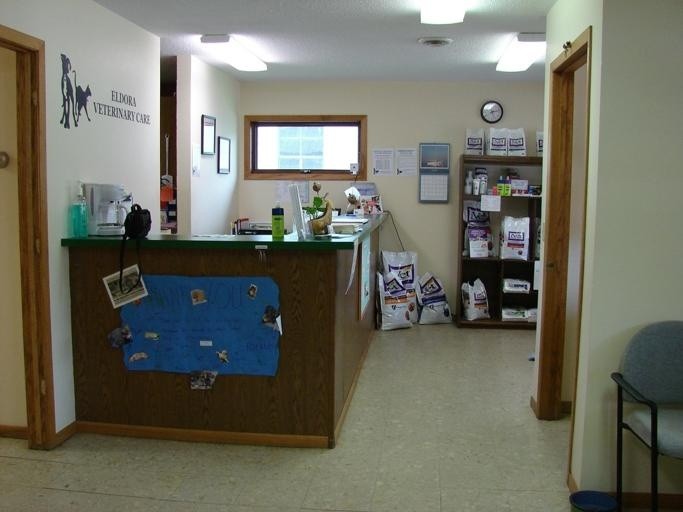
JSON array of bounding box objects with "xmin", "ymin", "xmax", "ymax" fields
[{"xmin": 479, "ymin": 99, "xmax": 503, "ymax": 123}]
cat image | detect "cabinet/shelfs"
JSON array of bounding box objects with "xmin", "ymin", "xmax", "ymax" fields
[{"xmin": 456, "ymin": 152, "xmax": 541, "ymax": 331}]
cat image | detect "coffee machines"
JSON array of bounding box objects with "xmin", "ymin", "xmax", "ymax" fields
[{"xmin": 80, "ymin": 182, "xmax": 133, "ymax": 237}]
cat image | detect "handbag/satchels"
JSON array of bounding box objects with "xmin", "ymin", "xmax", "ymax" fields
[{"xmin": 125, "ymin": 204, "xmax": 151, "ymax": 238}]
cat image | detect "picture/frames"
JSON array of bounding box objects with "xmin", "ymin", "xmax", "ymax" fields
[{"xmin": 199, "ymin": 114, "xmax": 231, "ymax": 175}]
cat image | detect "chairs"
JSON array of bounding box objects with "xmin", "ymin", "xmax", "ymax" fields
[{"xmin": 612, "ymin": 319, "xmax": 681, "ymax": 510}]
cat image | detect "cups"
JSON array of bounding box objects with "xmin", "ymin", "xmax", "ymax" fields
[{"xmin": 271, "ymin": 200, "xmax": 285, "ymax": 243}]
